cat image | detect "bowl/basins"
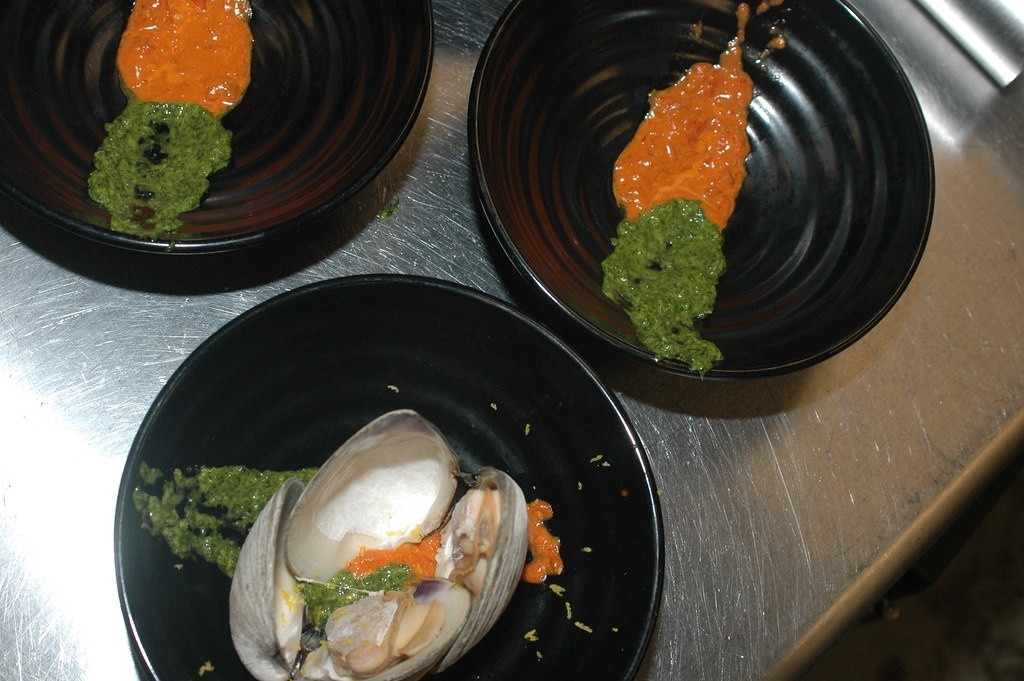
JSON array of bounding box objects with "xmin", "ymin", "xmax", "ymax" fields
[
  {"xmin": 111, "ymin": 272, "xmax": 665, "ymax": 681},
  {"xmin": 465, "ymin": 1, "xmax": 937, "ymax": 381},
  {"xmin": 0, "ymin": 0, "xmax": 434, "ymax": 256}
]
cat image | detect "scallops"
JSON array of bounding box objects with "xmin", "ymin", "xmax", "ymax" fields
[{"xmin": 227, "ymin": 408, "xmax": 529, "ymax": 681}]
